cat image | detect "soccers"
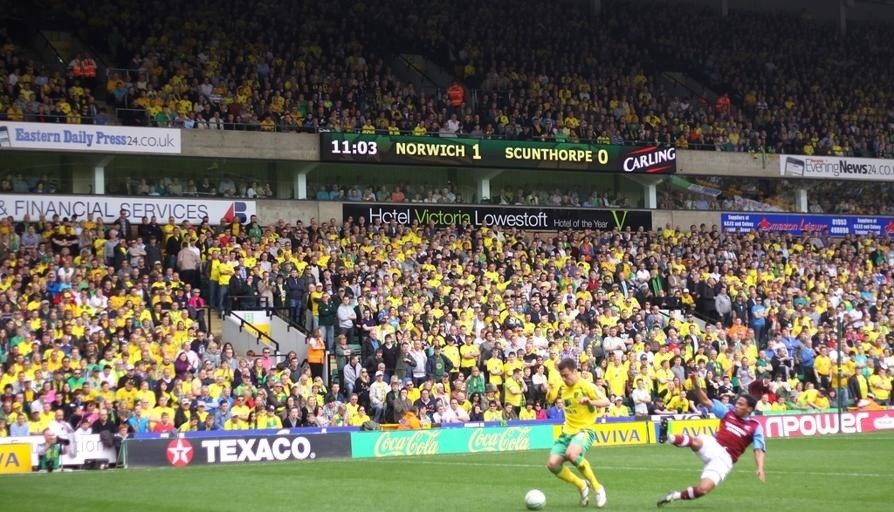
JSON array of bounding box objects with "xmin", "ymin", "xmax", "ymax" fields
[{"xmin": 525, "ymin": 491, "xmax": 546, "ymax": 508}]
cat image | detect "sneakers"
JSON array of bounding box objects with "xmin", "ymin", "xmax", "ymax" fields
[
  {"xmin": 657, "ymin": 490, "xmax": 677, "ymax": 507},
  {"xmin": 594, "ymin": 485, "xmax": 607, "ymax": 508},
  {"xmin": 579, "ymin": 480, "xmax": 591, "ymax": 507},
  {"xmin": 659, "ymin": 418, "xmax": 668, "ymax": 443}
]
